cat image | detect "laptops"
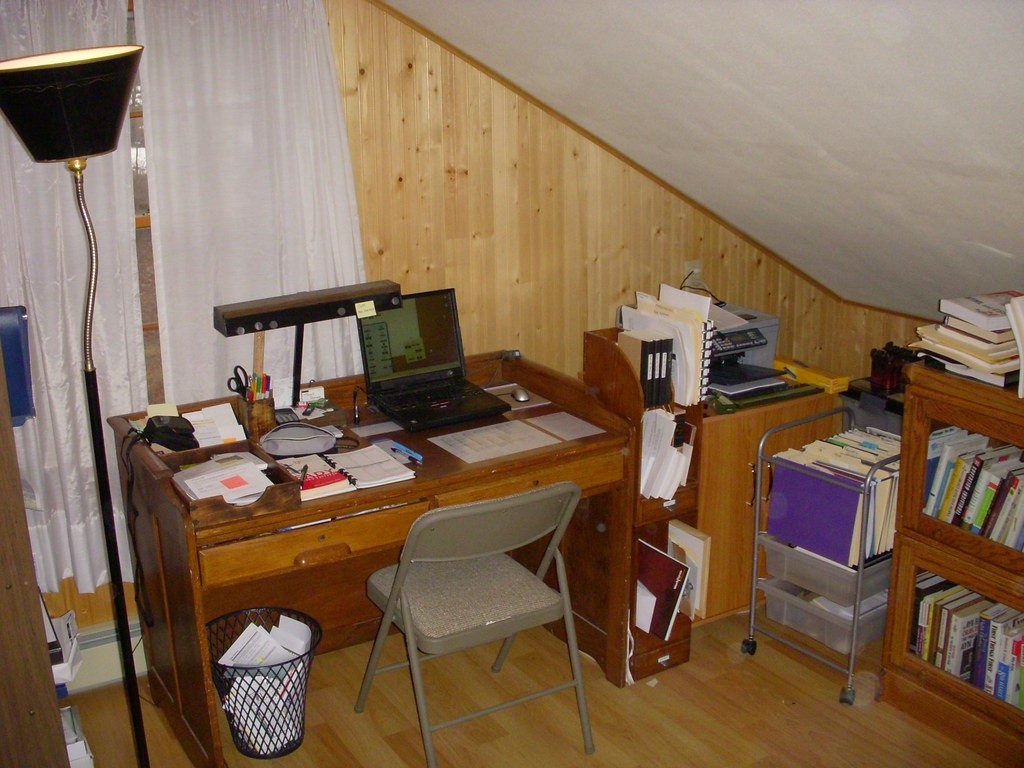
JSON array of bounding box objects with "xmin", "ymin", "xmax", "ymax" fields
[{"xmin": 356, "ymin": 288, "xmax": 512, "ymax": 433}]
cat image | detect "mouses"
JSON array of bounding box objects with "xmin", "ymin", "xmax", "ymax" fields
[{"xmin": 511, "ymin": 388, "xmax": 530, "ymax": 402}]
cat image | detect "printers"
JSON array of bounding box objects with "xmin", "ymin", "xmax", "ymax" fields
[{"xmin": 708, "ymin": 301, "xmax": 787, "ymax": 399}]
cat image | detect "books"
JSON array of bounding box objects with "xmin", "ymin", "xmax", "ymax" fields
[
  {"xmin": 617, "ymin": 282, "xmax": 1024, "ymax": 713},
  {"xmin": 173, "ymin": 444, "xmax": 418, "ymax": 507}
]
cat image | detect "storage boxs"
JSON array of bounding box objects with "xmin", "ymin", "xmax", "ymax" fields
[
  {"xmin": 773, "ymin": 352, "xmax": 850, "ymax": 395},
  {"xmin": 756, "ymin": 533, "xmax": 892, "ymax": 606},
  {"xmin": 758, "ymin": 578, "xmax": 887, "ymax": 655},
  {"xmin": 35, "ymin": 584, "xmax": 95, "ymax": 768}
]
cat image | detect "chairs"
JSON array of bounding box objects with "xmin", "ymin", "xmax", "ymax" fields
[{"xmin": 349, "ymin": 477, "xmax": 596, "ymax": 768}]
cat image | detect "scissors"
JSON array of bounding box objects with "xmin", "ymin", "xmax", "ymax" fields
[{"xmin": 227, "ymin": 364, "xmax": 248, "ymax": 398}]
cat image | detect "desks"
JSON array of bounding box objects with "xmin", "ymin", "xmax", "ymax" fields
[{"xmin": 116, "ymin": 348, "xmax": 640, "ymax": 768}]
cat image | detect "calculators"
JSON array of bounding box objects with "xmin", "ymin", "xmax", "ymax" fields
[{"xmin": 275, "ymin": 408, "xmax": 299, "ymax": 425}]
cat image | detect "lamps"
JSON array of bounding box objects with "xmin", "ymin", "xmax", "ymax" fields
[
  {"xmin": 0, "ymin": 46, "xmax": 153, "ymax": 768},
  {"xmin": 211, "ymin": 280, "xmax": 405, "ymax": 406}
]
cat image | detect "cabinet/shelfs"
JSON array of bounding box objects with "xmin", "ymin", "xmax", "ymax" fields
[
  {"xmin": 582, "ymin": 327, "xmax": 703, "ymax": 687},
  {"xmin": 742, "ymin": 408, "xmax": 902, "ymax": 705},
  {"xmin": 875, "ymin": 361, "xmax": 1024, "ymax": 768},
  {"xmin": 692, "ymin": 379, "xmax": 846, "ymax": 626}
]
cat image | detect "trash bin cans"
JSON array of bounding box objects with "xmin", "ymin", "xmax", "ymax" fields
[{"xmin": 201, "ymin": 605, "xmax": 323, "ymax": 760}]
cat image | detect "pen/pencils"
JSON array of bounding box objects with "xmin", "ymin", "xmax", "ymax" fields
[
  {"xmin": 390, "ymin": 447, "xmax": 423, "ymax": 466},
  {"xmin": 353, "ymin": 390, "xmax": 360, "ymax": 424},
  {"xmin": 298, "ymin": 464, "xmax": 308, "ymax": 490},
  {"xmin": 247, "ymin": 372, "xmax": 271, "ymax": 402}
]
